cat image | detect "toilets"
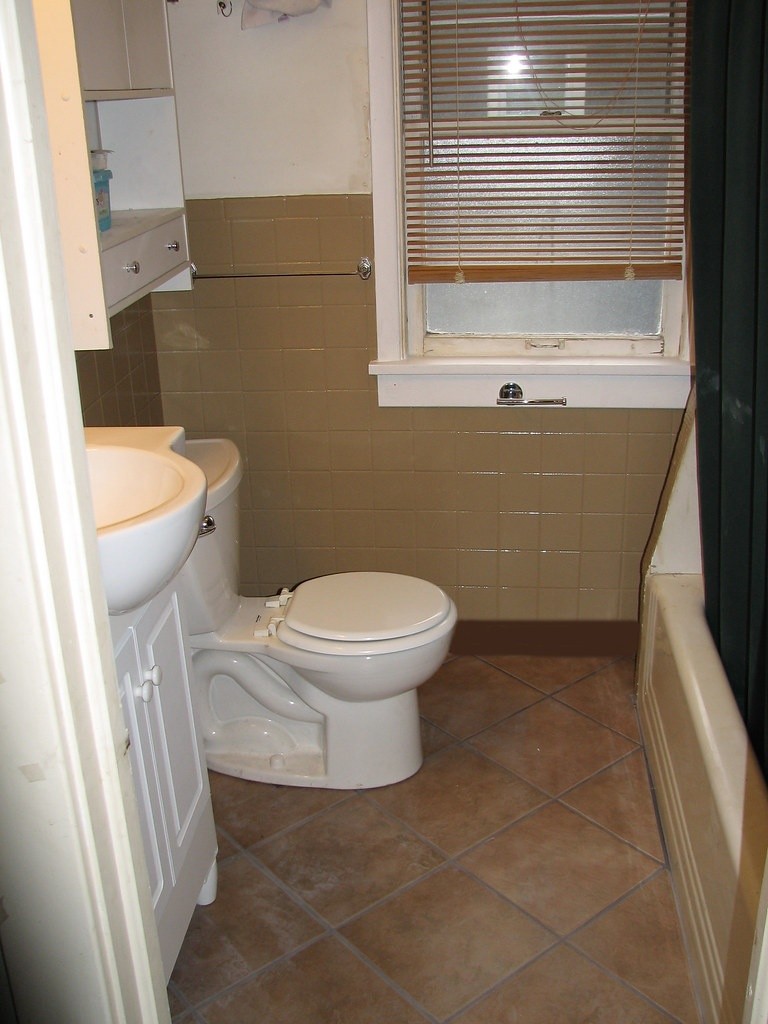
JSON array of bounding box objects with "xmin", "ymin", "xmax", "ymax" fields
[{"xmin": 173, "ymin": 439, "xmax": 457, "ymax": 788}]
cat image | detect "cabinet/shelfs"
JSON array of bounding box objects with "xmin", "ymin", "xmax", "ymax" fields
[
  {"xmin": 105, "ymin": 566, "xmax": 219, "ymax": 984},
  {"xmin": 34, "ymin": 0, "xmax": 192, "ymax": 352}
]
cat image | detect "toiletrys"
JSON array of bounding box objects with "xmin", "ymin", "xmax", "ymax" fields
[{"xmin": 90, "ymin": 145, "xmax": 112, "ymax": 233}]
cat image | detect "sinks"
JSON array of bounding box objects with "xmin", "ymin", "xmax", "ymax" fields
[{"xmin": 84, "ymin": 425, "xmax": 207, "ymax": 617}]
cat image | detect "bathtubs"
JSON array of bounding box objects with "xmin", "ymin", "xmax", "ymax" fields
[{"xmin": 632, "ymin": 570, "xmax": 768, "ymax": 1024}]
